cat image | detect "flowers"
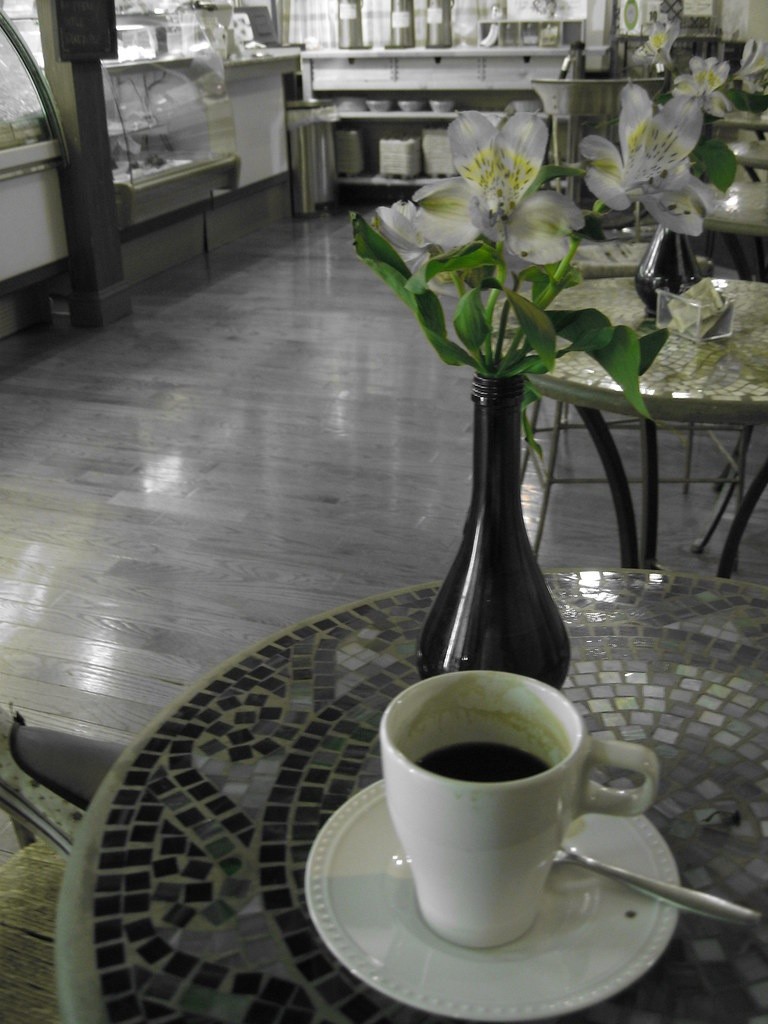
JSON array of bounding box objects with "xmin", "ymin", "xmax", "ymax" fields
[{"xmin": 348, "ymin": 32, "xmax": 768, "ymax": 457}]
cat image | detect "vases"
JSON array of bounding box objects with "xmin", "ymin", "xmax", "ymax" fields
[
  {"xmin": 415, "ymin": 376, "xmax": 573, "ymax": 690},
  {"xmin": 635, "ymin": 224, "xmax": 704, "ymax": 319}
]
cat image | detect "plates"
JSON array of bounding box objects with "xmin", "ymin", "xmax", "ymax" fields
[
  {"xmin": 333, "ymin": 129, "xmax": 364, "ymax": 178},
  {"xmin": 304, "ymin": 776, "xmax": 682, "ymax": 1023},
  {"xmin": 377, "ymin": 125, "xmax": 458, "ymax": 179}
]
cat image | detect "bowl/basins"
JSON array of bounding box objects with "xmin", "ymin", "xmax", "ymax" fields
[
  {"xmin": 398, "ymin": 100, "xmax": 424, "ymax": 111},
  {"xmin": 365, "ymin": 99, "xmax": 391, "ymax": 111},
  {"xmin": 429, "ymin": 99, "xmax": 455, "ymax": 113}
]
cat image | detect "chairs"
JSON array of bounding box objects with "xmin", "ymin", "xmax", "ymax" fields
[
  {"xmin": 0, "ymin": 697, "xmax": 130, "ymax": 1024},
  {"xmin": 570, "ymin": 242, "xmax": 713, "ymax": 286}
]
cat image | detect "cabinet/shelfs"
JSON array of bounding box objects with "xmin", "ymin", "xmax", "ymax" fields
[{"xmin": 300, "ymin": 45, "xmax": 608, "ymax": 187}]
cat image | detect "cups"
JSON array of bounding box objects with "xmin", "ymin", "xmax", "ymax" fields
[{"xmin": 377, "ymin": 670, "xmax": 662, "ymax": 949}]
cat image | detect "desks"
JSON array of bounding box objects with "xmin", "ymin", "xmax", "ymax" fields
[
  {"xmin": 54, "ymin": 564, "xmax": 768, "ymax": 1024},
  {"xmin": 480, "ymin": 278, "xmax": 768, "ymax": 576},
  {"xmin": 689, "ymin": 111, "xmax": 768, "ymax": 280}
]
